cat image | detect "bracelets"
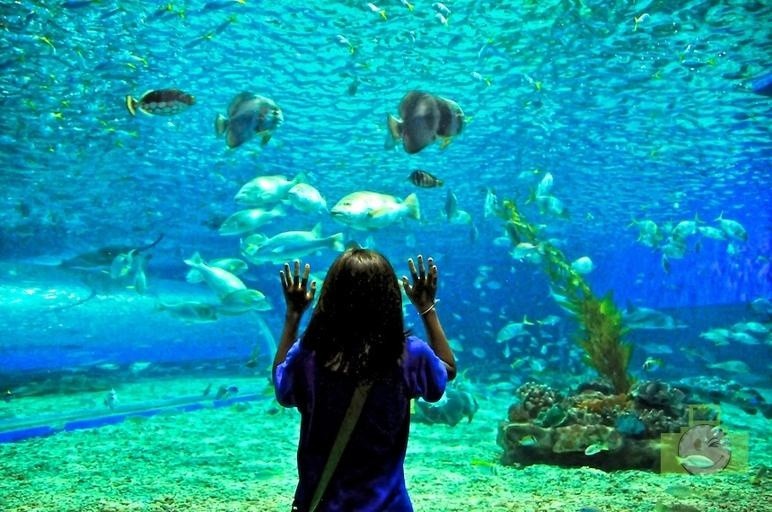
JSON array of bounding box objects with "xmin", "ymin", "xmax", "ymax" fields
[{"xmin": 418, "ymin": 304, "xmax": 435, "ymax": 317}]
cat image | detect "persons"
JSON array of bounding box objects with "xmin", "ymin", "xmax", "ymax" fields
[{"xmin": 269, "ymin": 244, "xmax": 459, "ymax": 512}]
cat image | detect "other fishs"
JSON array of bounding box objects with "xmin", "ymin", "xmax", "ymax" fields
[{"xmin": 0, "ymin": 1, "xmax": 771, "ymax": 472}]
